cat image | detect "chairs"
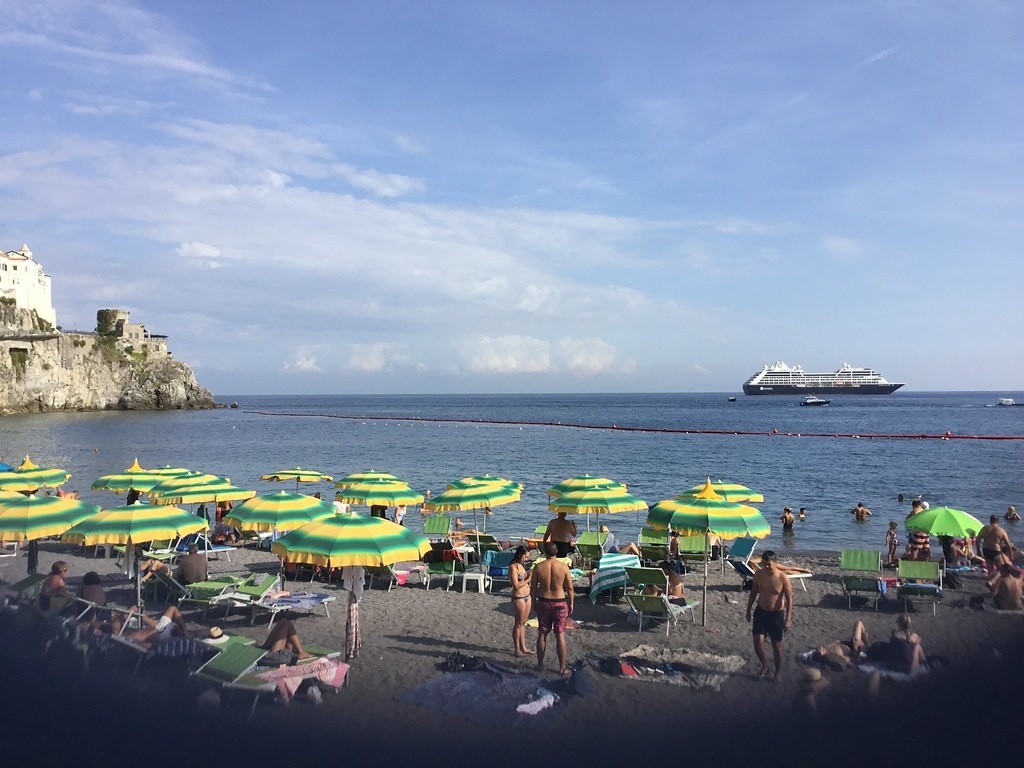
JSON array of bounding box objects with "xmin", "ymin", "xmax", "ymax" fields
[{"xmin": 0, "ymin": 516, "xmax": 1024, "ymax": 722}]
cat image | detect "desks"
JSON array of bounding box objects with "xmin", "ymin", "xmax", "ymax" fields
[{"xmin": 589, "ymin": 552, "xmax": 642, "ymax": 605}]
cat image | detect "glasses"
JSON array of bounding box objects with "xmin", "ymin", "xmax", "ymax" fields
[{"xmin": 62, "ymin": 569, "xmax": 67, "ymax": 572}]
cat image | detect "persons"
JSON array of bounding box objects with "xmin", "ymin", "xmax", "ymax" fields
[
  {"xmin": 217, "ymin": 501, "xmax": 236, "ymax": 542},
  {"xmin": 392, "ymin": 505, "xmax": 406, "ymax": 523},
  {"xmin": 486, "ymin": 507, "xmax": 492, "ymax": 515},
  {"xmin": 333, "ymin": 495, "xmax": 350, "ymax": 513},
  {"xmin": 81, "ymin": 571, "xmax": 105, "ymax": 606},
  {"xmin": 456, "ymin": 518, "xmax": 462, "ymax": 530},
  {"xmin": 138, "ymin": 558, "xmax": 180, "ymax": 584},
  {"xmin": 66, "ymin": 490, "xmax": 78, "ymax": 499},
  {"xmin": 643, "ymin": 585, "xmax": 661, "ymax": 595},
  {"xmin": 425, "ymin": 490, "xmax": 430, "ymax": 498},
  {"xmin": 747, "ymin": 550, "xmax": 814, "ymax": 683},
  {"xmin": 601, "ymin": 525, "xmax": 642, "ymax": 556},
  {"xmin": 709, "ymin": 532, "xmax": 720, "ymax": 545},
  {"xmin": 1, "ymin": 540, "xmax": 27, "ymax": 550},
  {"xmin": 176, "ymin": 544, "xmax": 207, "ymax": 584},
  {"xmin": 419, "ymin": 503, "xmax": 432, "ymax": 513},
  {"xmin": 125, "ymin": 607, "xmax": 194, "ymax": 648},
  {"xmin": 659, "ymin": 562, "xmax": 682, "ymax": 598},
  {"xmin": 542, "ymin": 512, "xmax": 577, "ymax": 559},
  {"xmin": 670, "ymin": 531, "xmax": 680, "ymax": 545},
  {"xmin": 508, "ymin": 546, "xmax": 536, "ymax": 657},
  {"xmin": 88, "ymin": 605, "xmax": 138, "ymax": 637},
  {"xmin": 256, "ymin": 619, "xmax": 313, "ymax": 666},
  {"xmin": 802, "ymin": 614, "xmax": 924, "ymax": 714},
  {"xmin": 315, "ymin": 493, "xmax": 322, "ymax": 499},
  {"xmin": 40, "ymin": 560, "xmax": 76, "ymax": 610},
  {"xmin": 779, "ymin": 493, "xmax": 1024, "ymax": 610},
  {"xmin": 462, "ymin": 529, "xmax": 476, "ymax": 534},
  {"xmin": 531, "ymin": 542, "xmax": 574, "ymax": 675}
]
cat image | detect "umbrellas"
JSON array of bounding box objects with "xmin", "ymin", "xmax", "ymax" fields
[
  {"xmin": 0, "ymin": 453, "xmax": 770, "ymax": 686},
  {"xmin": 904, "ymin": 506, "xmax": 985, "ymax": 538}
]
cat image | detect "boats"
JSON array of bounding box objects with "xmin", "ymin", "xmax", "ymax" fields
[
  {"xmin": 833, "ymin": 381, "xmax": 844, "ymax": 386},
  {"xmin": 798, "ymin": 399, "xmax": 831, "ymax": 407},
  {"xmin": 845, "ymin": 381, "xmax": 852, "ymax": 386},
  {"xmin": 728, "ymin": 396, "xmax": 736, "ymax": 401}
]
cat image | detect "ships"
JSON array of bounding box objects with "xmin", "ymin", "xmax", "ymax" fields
[{"xmin": 741, "ymin": 360, "xmax": 907, "ymax": 397}]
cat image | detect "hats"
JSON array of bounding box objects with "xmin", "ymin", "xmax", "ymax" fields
[
  {"xmin": 202, "ymin": 626, "xmax": 229, "ymax": 644},
  {"xmin": 796, "ymin": 668, "xmax": 830, "ymax": 691}
]
